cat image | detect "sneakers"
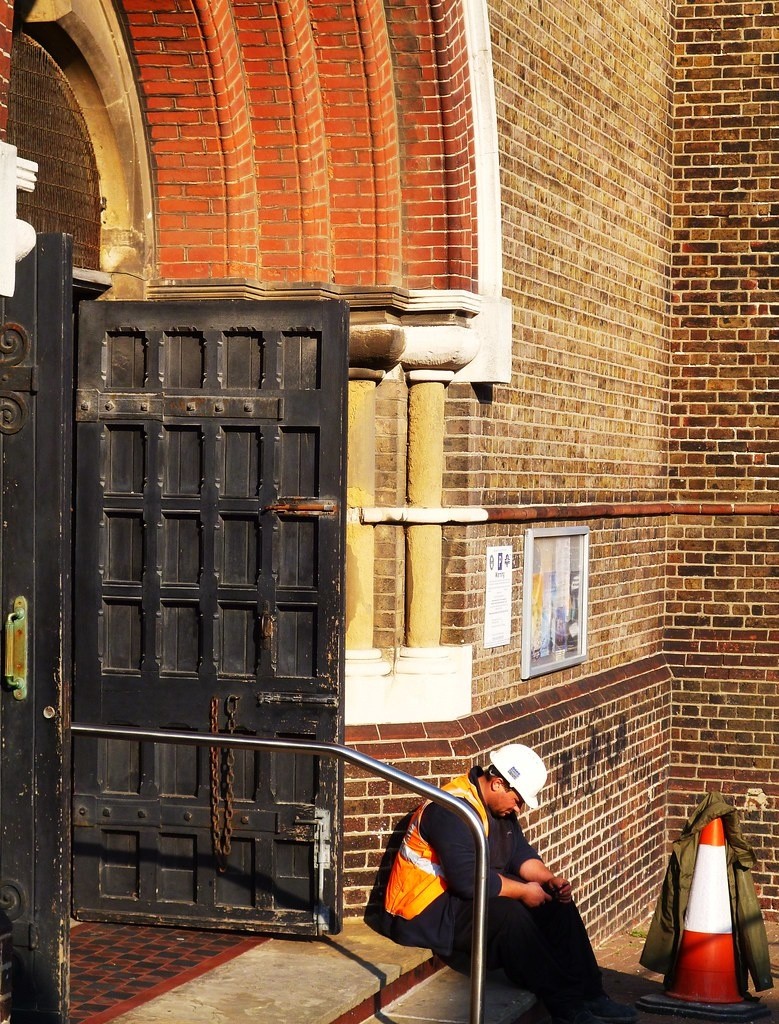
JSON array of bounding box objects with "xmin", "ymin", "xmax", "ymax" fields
[{"xmin": 551, "ymin": 994, "xmax": 640, "ymax": 1024}]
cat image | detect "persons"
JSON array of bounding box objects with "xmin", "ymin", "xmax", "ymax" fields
[{"xmin": 383, "ymin": 743, "xmax": 638, "ymax": 1023}]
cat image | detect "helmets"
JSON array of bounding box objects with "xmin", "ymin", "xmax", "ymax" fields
[{"xmin": 490, "ymin": 743, "xmax": 547, "ymax": 808}]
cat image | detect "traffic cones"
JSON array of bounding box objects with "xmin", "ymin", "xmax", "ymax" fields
[{"xmin": 632, "ymin": 815, "xmax": 770, "ymax": 1023}]
